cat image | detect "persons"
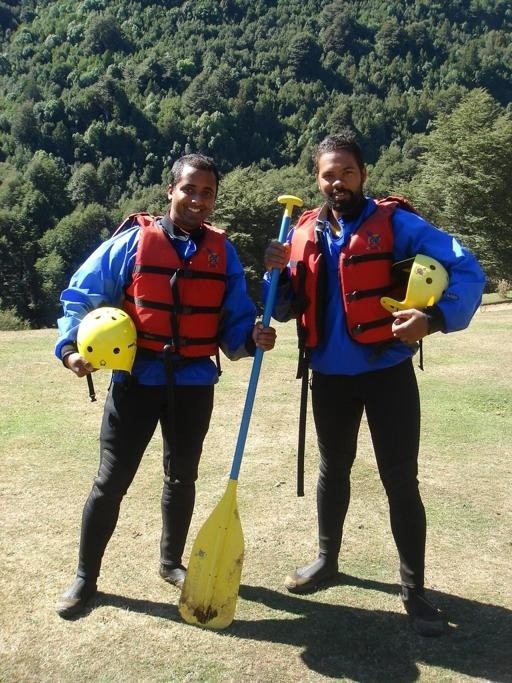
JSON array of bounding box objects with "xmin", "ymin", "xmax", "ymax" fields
[
  {"xmin": 52, "ymin": 152, "xmax": 279, "ymax": 621},
  {"xmin": 261, "ymin": 134, "xmax": 488, "ymax": 639}
]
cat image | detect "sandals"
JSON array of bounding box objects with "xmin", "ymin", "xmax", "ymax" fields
[
  {"xmin": 57, "ymin": 572, "xmax": 96, "ymax": 617},
  {"xmin": 284, "ymin": 555, "xmax": 338, "ymax": 591},
  {"xmin": 401, "ymin": 583, "xmax": 444, "ymax": 637},
  {"xmin": 158, "ymin": 557, "xmax": 186, "ymax": 590}
]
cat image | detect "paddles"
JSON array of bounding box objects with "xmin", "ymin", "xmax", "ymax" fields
[{"xmin": 178, "ymin": 194, "xmax": 304, "ymax": 628}]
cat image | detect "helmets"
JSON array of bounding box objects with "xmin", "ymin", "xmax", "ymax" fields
[
  {"xmin": 380, "ymin": 253, "xmax": 450, "ymax": 313},
  {"xmin": 76, "ymin": 307, "xmax": 137, "ymax": 376}
]
